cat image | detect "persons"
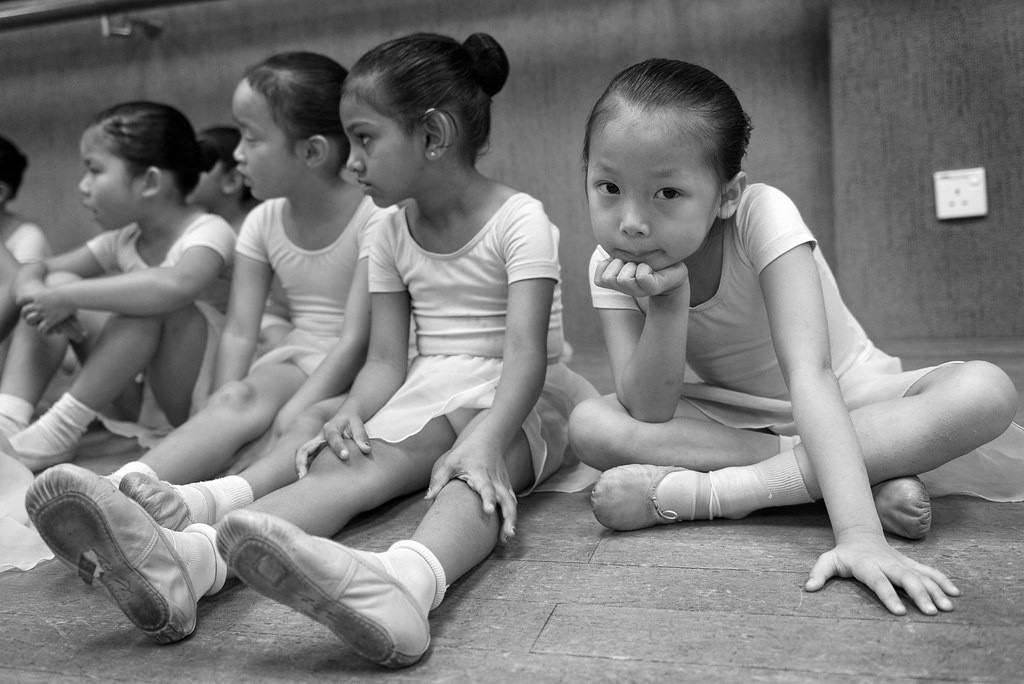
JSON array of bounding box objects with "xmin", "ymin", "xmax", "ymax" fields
[
  {"xmin": 569, "ymin": 59, "xmax": 1019, "ymax": 617},
  {"xmin": 1, "ymin": 100, "xmax": 265, "ymax": 474},
  {"xmin": 26, "ymin": 30, "xmax": 605, "ymax": 668},
  {"xmin": 107, "ymin": 49, "xmax": 399, "ymax": 533}
]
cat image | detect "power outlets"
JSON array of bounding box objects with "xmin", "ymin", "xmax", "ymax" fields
[{"xmin": 933, "ymin": 167, "xmax": 988, "ymax": 220}]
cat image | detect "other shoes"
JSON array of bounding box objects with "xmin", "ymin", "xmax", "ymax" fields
[
  {"xmin": 119, "ymin": 473, "xmax": 216, "ymax": 532},
  {"xmin": 869, "ymin": 473, "xmax": 932, "ymax": 539},
  {"xmin": 591, "ymin": 462, "xmax": 711, "ymax": 531},
  {"xmin": 24, "ymin": 464, "xmax": 196, "ymax": 643},
  {"xmin": 215, "ymin": 510, "xmax": 431, "ymax": 667},
  {"xmin": 0, "ymin": 432, "xmax": 80, "ymax": 472}
]
